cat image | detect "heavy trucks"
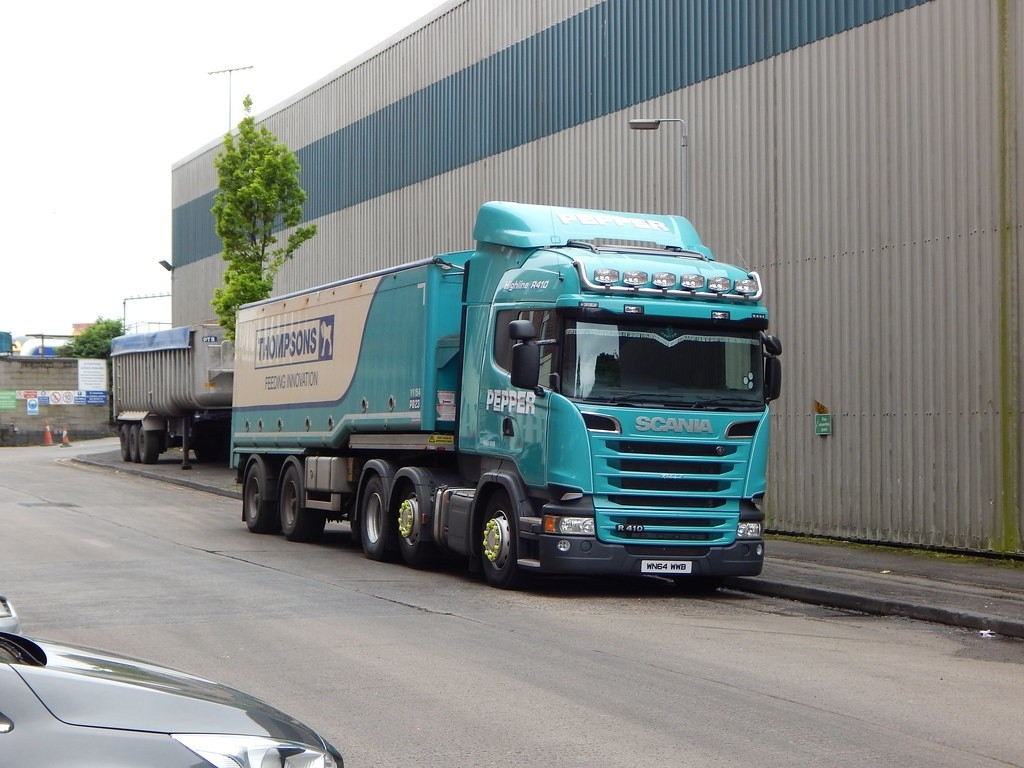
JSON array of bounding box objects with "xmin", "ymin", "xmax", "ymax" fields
[{"xmin": 236, "ymin": 201, "xmax": 784, "ymax": 595}]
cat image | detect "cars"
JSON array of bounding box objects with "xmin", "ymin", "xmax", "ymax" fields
[{"xmin": 0, "ymin": 597, "xmax": 345, "ymax": 768}]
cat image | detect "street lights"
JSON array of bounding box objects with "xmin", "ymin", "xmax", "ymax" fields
[{"xmin": 627, "ymin": 116, "xmax": 690, "ymax": 219}]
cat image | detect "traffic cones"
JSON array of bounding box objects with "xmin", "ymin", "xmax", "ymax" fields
[
  {"xmin": 61, "ymin": 425, "xmax": 72, "ymax": 447},
  {"xmin": 43, "ymin": 420, "xmax": 54, "ymax": 446}
]
